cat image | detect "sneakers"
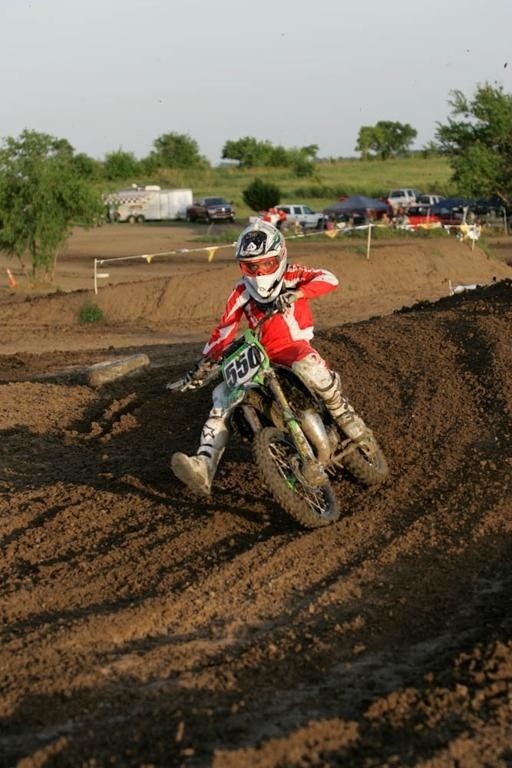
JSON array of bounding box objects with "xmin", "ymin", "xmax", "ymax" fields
[
  {"xmin": 326, "ymin": 394, "xmax": 368, "ymax": 444},
  {"xmin": 170, "ymin": 448, "xmax": 217, "ymax": 499}
]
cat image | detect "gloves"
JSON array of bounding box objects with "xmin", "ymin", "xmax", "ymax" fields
[
  {"xmin": 271, "ymin": 292, "xmax": 296, "ymax": 314},
  {"xmin": 182, "ymin": 369, "xmax": 205, "ymax": 392}
]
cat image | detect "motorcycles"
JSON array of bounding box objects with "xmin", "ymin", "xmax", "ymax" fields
[{"xmin": 167, "ymin": 293, "xmax": 389, "ymax": 529}]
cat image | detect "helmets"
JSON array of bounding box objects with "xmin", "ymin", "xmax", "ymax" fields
[{"xmin": 234, "ymin": 219, "xmax": 290, "ymax": 299}]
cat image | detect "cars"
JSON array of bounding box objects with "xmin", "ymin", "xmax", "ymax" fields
[
  {"xmin": 186, "ymin": 197, "xmax": 236, "ymax": 224},
  {"xmin": 384, "ymin": 189, "xmax": 512, "ymax": 220}
]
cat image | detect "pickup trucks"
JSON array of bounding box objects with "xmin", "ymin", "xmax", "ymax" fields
[{"xmin": 249, "ymin": 205, "xmax": 329, "ymax": 231}]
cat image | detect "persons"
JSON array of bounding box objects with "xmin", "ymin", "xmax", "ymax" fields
[
  {"xmin": 458, "ymin": 217, "xmax": 481, "ymax": 242},
  {"xmin": 170, "ymin": 217, "xmax": 368, "ymax": 496},
  {"xmin": 259, "ymin": 207, "xmax": 408, "ymax": 234}
]
